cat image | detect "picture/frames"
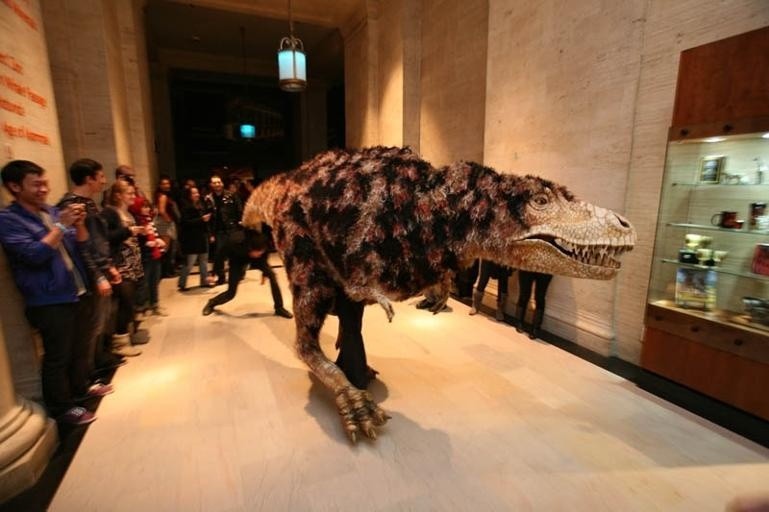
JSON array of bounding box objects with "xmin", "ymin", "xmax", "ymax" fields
[{"xmin": 696, "ymin": 154, "xmax": 726, "ymax": 185}]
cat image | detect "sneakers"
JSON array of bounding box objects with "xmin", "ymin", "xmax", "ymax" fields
[
  {"xmin": 75, "ymin": 384, "xmax": 114, "ymax": 401},
  {"xmin": 62, "ymin": 406, "xmax": 97, "ymax": 426}
]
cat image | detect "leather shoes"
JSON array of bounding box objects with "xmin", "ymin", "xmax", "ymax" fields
[
  {"xmin": 203, "ymin": 298, "xmax": 214, "ymax": 316},
  {"xmin": 275, "ymin": 307, "xmax": 293, "ymax": 318}
]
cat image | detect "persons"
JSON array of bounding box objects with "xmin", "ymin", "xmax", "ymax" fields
[
  {"xmin": 53, "ymin": 158, "xmax": 165, "ymax": 374},
  {"xmin": 151, "ymin": 167, "xmax": 293, "ymax": 318},
  {"xmin": 0, "ymin": 160, "xmax": 114, "ymax": 426},
  {"xmin": 414, "ymin": 258, "xmax": 554, "ymax": 340}
]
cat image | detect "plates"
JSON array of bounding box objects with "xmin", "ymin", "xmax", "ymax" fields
[{"xmin": 749, "ymin": 228, "xmax": 769, "ymax": 234}]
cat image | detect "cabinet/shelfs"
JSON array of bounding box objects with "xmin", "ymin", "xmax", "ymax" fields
[{"xmin": 638, "ymin": 25, "xmax": 769, "ymax": 421}]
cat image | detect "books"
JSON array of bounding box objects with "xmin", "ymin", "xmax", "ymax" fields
[{"xmin": 672, "ymin": 267, "xmax": 719, "ymax": 309}]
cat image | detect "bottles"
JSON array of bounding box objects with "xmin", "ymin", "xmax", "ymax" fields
[
  {"xmin": 705, "ymin": 271, "xmax": 719, "ymax": 310},
  {"xmin": 754, "ymin": 163, "xmax": 764, "ymax": 185}
]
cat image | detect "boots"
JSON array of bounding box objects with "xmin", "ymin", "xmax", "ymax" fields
[
  {"xmin": 128, "ymin": 323, "xmax": 149, "ymax": 344},
  {"xmin": 111, "ymin": 333, "xmax": 143, "ymax": 357}
]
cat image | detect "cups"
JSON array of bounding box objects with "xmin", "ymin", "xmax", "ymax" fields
[
  {"xmin": 754, "ymin": 216, "xmax": 768, "ymax": 229},
  {"xmin": 710, "ymin": 210, "xmax": 738, "ymax": 228},
  {"xmin": 733, "ymin": 219, "xmax": 744, "ymax": 228}
]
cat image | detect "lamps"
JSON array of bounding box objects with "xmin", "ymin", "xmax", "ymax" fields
[{"xmin": 275, "ymin": 0, "xmax": 308, "ymax": 93}]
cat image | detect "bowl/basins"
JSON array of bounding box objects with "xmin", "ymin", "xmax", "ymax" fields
[{"xmin": 742, "ymin": 296, "xmax": 769, "ymax": 324}]
